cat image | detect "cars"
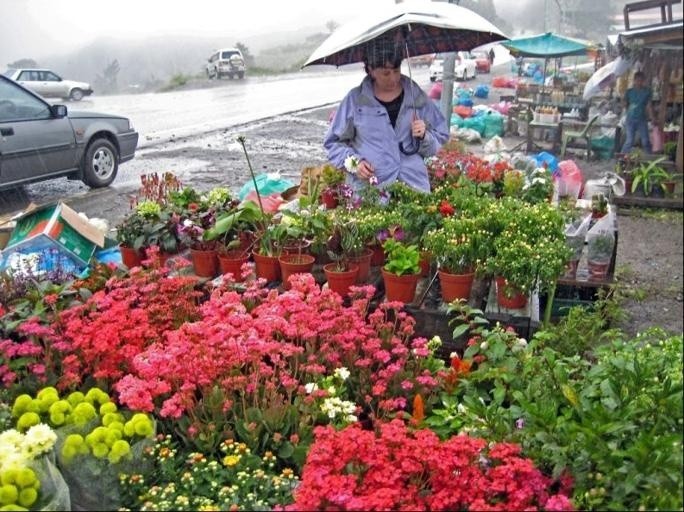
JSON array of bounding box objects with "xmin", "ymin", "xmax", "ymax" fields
[
  {"xmin": 407, "ymin": 52, "xmax": 434, "ymax": 69},
  {"xmin": 471, "ymin": 49, "xmax": 492, "ymax": 74},
  {"xmin": 429, "ymin": 49, "xmax": 478, "ymax": 81},
  {"xmin": 0, "ymin": 72, "xmax": 139, "ymax": 196},
  {"xmin": 1, "ymin": 67, "xmax": 94, "ymax": 102}
]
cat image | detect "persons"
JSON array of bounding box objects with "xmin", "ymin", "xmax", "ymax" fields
[
  {"xmin": 322, "ymin": 41, "xmax": 447, "ymax": 199},
  {"xmin": 530, "ymin": 50, "xmax": 667, "ymax": 157},
  {"xmin": 488, "ymin": 48, "xmax": 495, "ymax": 64}
]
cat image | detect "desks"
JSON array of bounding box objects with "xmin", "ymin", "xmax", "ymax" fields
[
  {"xmin": 515, "ymin": 97, "xmax": 589, "ymax": 119},
  {"xmin": 525, "ymin": 118, "xmax": 562, "ymax": 152}
]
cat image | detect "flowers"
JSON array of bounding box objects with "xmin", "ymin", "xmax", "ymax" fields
[{"xmin": 0, "ymin": 245, "xmax": 684, "ymax": 510}]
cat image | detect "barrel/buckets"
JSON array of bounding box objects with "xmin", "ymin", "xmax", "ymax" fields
[
  {"xmin": 583, "ymin": 178, "xmax": 613, "ymax": 201},
  {"xmin": 92, "ymin": 244, "xmax": 122, "ymax": 264}
]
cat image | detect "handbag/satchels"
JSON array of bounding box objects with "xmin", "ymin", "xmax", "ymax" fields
[{"xmin": 651, "ymin": 124, "xmax": 664, "ymax": 153}]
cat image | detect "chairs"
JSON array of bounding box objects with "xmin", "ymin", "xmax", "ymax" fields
[{"xmin": 562, "ymin": 114, "xmax": 599, "ymax": 159}]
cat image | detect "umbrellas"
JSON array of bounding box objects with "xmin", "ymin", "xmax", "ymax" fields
[
  {"xmin": 297, "ymin": 1, "xmax": 511, "ymax": 126},
  {"xmin": 501, "ymin": 30, "xmax": 596, "ymax": 89}
]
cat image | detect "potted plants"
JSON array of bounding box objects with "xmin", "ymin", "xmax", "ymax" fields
[{"xmin": 119, "ymin": 154, "xmax": 682, "ymax": 310}]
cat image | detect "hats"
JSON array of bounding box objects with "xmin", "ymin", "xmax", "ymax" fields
[{"xmin": 596, "ymin": 99, "xmax": 608, "ymax": 109}]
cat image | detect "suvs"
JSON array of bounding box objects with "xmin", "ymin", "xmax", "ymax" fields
[{"xmin": 203, "ymin": 46, "xmax": 248, "ymax": 81}]
